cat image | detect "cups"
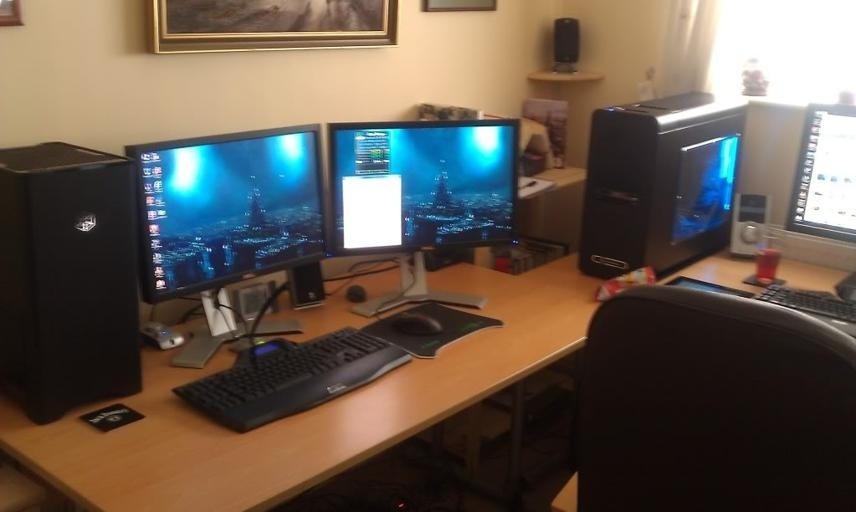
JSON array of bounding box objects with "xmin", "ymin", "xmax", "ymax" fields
[{"xmin": 755, "ymin": 224, "xmax": 786, "ymax": 285}]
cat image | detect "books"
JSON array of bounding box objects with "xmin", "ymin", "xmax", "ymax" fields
[
  {"xmin": 516, "ymin": 177, "xmax": 556, "ymax": 200},
  {"xmin": 519, "ymin": 97, "xmax": 569, "ymax": 170}
]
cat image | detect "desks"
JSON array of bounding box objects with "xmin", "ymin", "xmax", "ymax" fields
[{"xmin": 0, "ymin": 246, "xmax": 853, "ymax": 512}]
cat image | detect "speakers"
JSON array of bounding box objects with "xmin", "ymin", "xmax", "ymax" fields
[
  {"xmin": 730, "ymin": 191, "xmax": 774, "ymax": 258},
  {"xmin": 552, "ymin": 15, "xmax": 582, "ymax": 75},
  {"xmin": 286, "ymin": 260, "xmax": 326, "ymax": 307}
]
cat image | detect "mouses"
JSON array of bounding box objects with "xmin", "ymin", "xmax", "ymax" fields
[{"xmin": 400, "ymin": 311, "xmax": 443, "ymax": 336}]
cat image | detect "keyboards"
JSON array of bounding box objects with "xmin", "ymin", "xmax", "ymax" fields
[
  {"xmin": 756, "ymin": 284, "xmax": 855, "ymax": 324},
  {"xmin": 171, "ymin": 326, "xmax": 413, "ymax": 434}
]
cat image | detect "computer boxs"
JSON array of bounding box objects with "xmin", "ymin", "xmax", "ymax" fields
[
  {"xmin": 576, "ymin": 92, "xmax": 749, "ymax": 281},
  {"xmin": 1, "ymin": 140, "xmax": 144, "ymax": 426}
]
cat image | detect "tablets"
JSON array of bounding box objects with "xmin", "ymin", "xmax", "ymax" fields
[{"xmin": 663, "ymin": 275, "xmax": 756, "ymax": 299}]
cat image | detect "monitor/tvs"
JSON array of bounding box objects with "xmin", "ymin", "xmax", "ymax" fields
[
  {"xmin": 326, "ymin": 118, "xmax": 522, "ymax": 317},
  {"xmin": 781, "ymin": 102, "xmax": 856, "ymax": 306},
  {"xmin": 124, "ymin": 122, "xmax": 329, "ymax": 370}
]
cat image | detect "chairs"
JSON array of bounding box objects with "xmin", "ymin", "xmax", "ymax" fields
[{"xmin": 577, "ymin": 285, "xmax": 855, "ymax": 512}]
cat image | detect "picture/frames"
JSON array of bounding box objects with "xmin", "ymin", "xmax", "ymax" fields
[
  {"xmin": 143, "ymin": 0, "xmax": 400, "ymax": 55},
  {"xmin": 423, "ymin": 0, "xmax": 497, "ymax": 12}
]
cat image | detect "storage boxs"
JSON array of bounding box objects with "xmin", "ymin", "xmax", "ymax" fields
[{"xmin": 515, "ymin": 164, "xmax": 587, "ymax": 255}]
